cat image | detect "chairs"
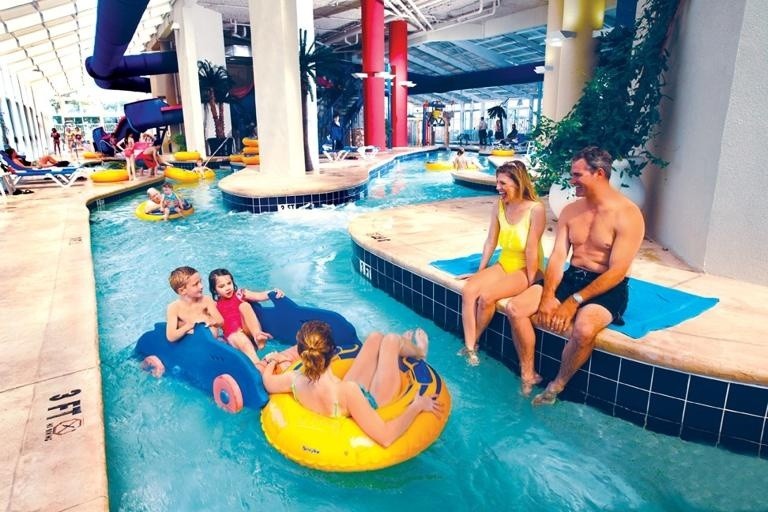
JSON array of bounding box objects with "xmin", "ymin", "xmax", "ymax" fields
[{"xmin": 0, "ymin": 148, "xmax": 84, "ymax": 191}]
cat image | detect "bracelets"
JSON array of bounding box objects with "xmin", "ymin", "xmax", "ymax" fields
[{"xmin": 573, "ymin": 292, "xmax": 584, "ymax": 305}]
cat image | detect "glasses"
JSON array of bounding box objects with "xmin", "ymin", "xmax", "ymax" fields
[{"xmin": 504, "ymin": 162, "xmax": 517, "ymax": 169}]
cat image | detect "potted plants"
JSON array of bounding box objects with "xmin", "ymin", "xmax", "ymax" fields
[
  {"xmin": 521, "ymin": 0, "xmax": 686, "ymax": 219},
  {"xmin": 197, "ymin": 59, "xmax": 237, "ymax": 166}
]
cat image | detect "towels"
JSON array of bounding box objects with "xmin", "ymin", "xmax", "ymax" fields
[{"xmin": 431, "ymin": 250, "xmax": 720, "ymax": 339}]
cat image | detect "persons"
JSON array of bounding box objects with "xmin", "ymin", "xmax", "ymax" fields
[
  {"xmin": 165, "ymin": 265, "xmax": 286, "ymax": 373},
  {"xmin": 453, "ymin": 145, "xmax": 470, "ymax": 173},
  {"xmin": 504, "ymin": 144, "xmax": 646, "ymax": 410},
  {"xmin": 453, "ymin": 159, "xmax": 547, "ymax": 367},
  {"xmin": 4, "ymin": 126, "xmax": 214, "ymax": 184},
  {"xmin": 261, "ymin": 319, "xmax": 445, "ymax": 448},
  {"xmin": 144, "ymin": 181, "xmax": 185, "ymax": 222},
  {"xmin": 477, "ymin": 116, "xmax": 518, "ymax": 152}
]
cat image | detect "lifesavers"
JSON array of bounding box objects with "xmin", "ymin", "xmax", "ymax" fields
[
  {"xmin": 427, "ymin": 159, "xmax": 478, "ymax": 173},
  {"xmin": 492, "ymin": 149, "xmax": 515, "ymax": 156},
  {"xmin": 83, "ymin": 152, "xmax": 100, "ymax": 158},
  {"xmin": 174, "ymin": 152, "xmax": 201, "ymax": 161},
  {"xmin": 90, "ymin": 170, "xmax": 129, "ymax": 182},
  {"xmin": 230, "ymin": 137, "xmax": 260, "ymax": 166},
  {"xmin": 200, "ymin": 170, "xmax": 214, "ymax": 179},
  {"xmin": 136, "ymin": 198, "xmax": 193, "ymax": 220},
  {"xmin": 261, "ymin": 345, "xmax": 452, "ymax": 472},
  {"xmin": 164, "ymin": 166, "xmax": 200, "ymax": 182}
]
